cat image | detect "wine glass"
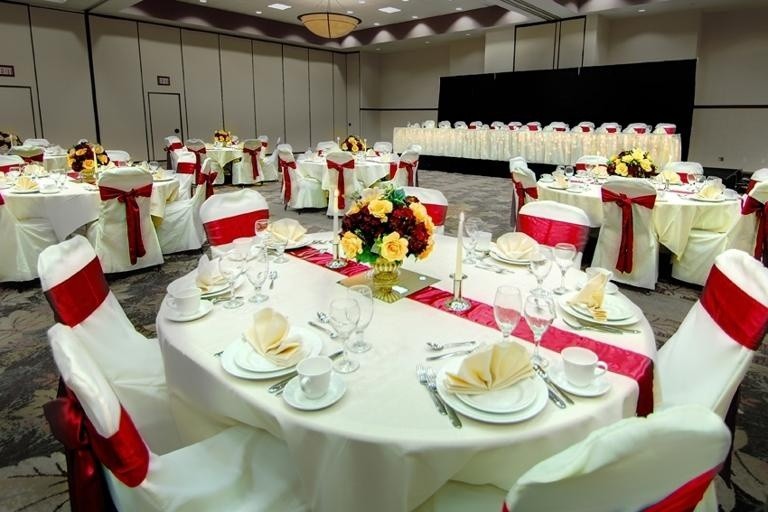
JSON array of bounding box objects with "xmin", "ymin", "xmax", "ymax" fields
[
  {"xmin": 219, "ymin": 219, "xmax": 290, "ymax": 309},
  {"xmin": 552, "ymin": 160, "xmax": 719, "ymax": 197},
  {"xmin": 328, "ymin": 284, "xmax": 374, "ymax": 373},
  {"xmin": 9, "ymin": 168, "xmax": 67, "ymax": 188},
  {"xmin": 95, "ymin": 159, "xmax": 159, "ymax": 181},
  {"xmin": 493, "ymin": 243, "xmax": 576, "ymax": 368},
  {"xmin": 305, "ymin": 145, "xmax": 390, "ymax": 164},
  {"xmin": 462, "ymin": 216, "xmax": 492, "ymax": 265}
]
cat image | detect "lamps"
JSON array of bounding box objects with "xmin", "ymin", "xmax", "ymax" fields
[{"xmin": 297, "ymin": 0, "xmax": 362, "ymax": 39}]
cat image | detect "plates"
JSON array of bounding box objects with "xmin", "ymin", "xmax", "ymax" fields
[
  {"xmin": 0, "ymin": 184, "xmax": 61, "ymax": 194},
  {"xmin": 161, "ymin": 234, "xmax": 348, "ymax": 410},
  {"xmin": 435, "ymin": 356, "xmax": 613, "ymax": 424},
  {"xmin": 153, "ymin": 176, "xmax": 174, "ymax": 181},
  {"xmin": 474, "ymin": 242, "xmax": 644, "ymax": 326},
  {"xmin": 539, "ymin": 178, "xmax": 737, "ymax": 202}
]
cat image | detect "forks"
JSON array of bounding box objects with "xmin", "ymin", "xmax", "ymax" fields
[{"xmin": 416, "ymin": 364, "xmax": 462, "ymax": 429}]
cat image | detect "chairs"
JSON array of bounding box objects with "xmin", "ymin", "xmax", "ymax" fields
[
  {"xmin": 0, "ymin": 121, "xmax": 448, "ymax": 282},
  {"xmin": 38, "ymin": 235, "xmax": 767, "ymax": 511},
  {"xmin": 408, "ymin": 121, "xmax": 677, "ymax": 133},
  {"xmin": 510, "ymin": 156, "xmax": 768, "ymax": 290}
]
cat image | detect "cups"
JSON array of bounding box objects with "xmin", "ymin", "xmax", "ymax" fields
[
  {"xmin": 561, "ymin": 346, "xmax": 608, "ymax": 388},
  {"xmin": 540, "ymin": 174, "xmax": 552, "ymax": 179}
]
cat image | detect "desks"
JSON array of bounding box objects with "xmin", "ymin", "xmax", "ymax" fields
[{"xmin": 157, "ymin": 229, "xmax": 655, "ymax": 448}]
